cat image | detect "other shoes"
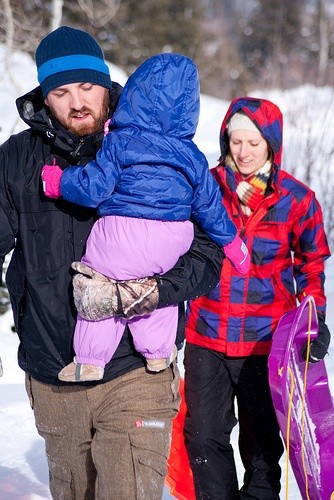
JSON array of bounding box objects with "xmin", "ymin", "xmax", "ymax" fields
[
  {"xmin": 146, "ymin": 344, "xmax": 178, "ymax": 371},
  {"xmin": 58, "ymin": 356, "xmax": 105, "ymax": 382}
]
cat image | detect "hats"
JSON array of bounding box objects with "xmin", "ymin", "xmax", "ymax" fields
[
  {"xmin": 226, "ymin": 110, "xmax": 262, "ymax": 136},
  {"xmin": 35, "ymin": 26, "xmax": 111, "ymax": 99}
]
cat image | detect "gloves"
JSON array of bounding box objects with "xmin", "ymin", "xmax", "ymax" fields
[
  {"xmin": 41, "ymin": 157, "xmax": 63, "ymax": 199},
  {"xmin": 303, "ymin": 318, "xmax": 331, "ymax": 363},
  {"xmin": 221, "ymin": 234, "xmax": 250, "ymax": 275},
  {"xmin": 71, "ymin": 261, "xmax": 159, "ymax": 321}
]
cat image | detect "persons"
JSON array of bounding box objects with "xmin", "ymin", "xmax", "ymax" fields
[
  {"xmin": 0, "ymin": 26, "xmax": 225, "ymax": 500},
  {"xmin": 41, "ymin": 52, "xmax": 250, "ymax": 381},
  {"xmin": 181, "ymin": 97, "xmax": 331, "ymax": 500}
]
